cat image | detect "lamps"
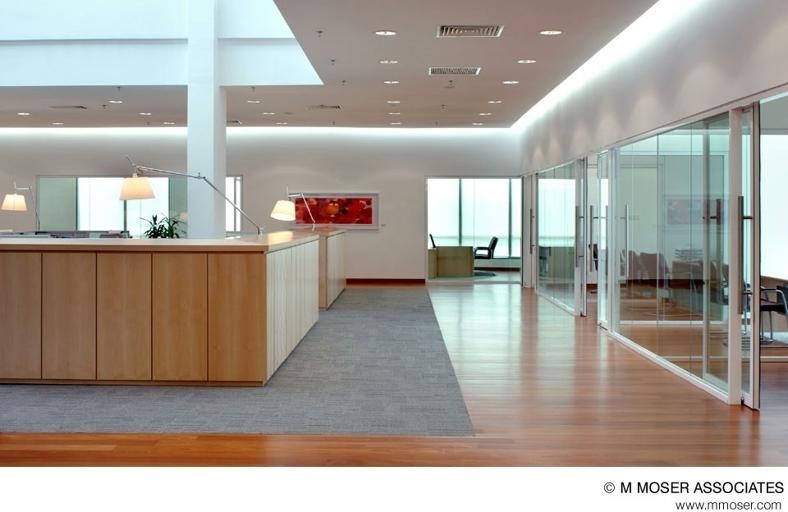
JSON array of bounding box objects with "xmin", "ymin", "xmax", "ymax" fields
[
  {"xmin": 269, "ymin": 186, "xmax": 316, "ymax": 226},
  {"xmin": 1, "ymin": 180, "xmax": 41, "ymax": 230},
  {"xmin": 119, "ymin": 155, "xmax": 264, "ymax": 236}
]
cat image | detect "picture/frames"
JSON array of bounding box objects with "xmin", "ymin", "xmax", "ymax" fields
[
  {"xmin": 287, "ymin": 192, "xmax": 379, "ymax": 231},
  {"xmin": 658, "ymin": 194, "xmax": 728, "ymax": 233}
]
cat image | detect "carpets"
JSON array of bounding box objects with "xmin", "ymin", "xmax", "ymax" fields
[{"xmin": 0, "ymin": 286, "xmax": 478, "ymax": 437}]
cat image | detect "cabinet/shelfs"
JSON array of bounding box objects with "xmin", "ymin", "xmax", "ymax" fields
[{"xmin": 428, "ymin": 244, "xmax": 474, "ymax": 277}]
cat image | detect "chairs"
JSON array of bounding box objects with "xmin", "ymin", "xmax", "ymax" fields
[
  {"xmin": 584, "ymin": 241, "xmax": 787, "ymax": 349},
  {"xmin": 473, "ymin": 236, "xmax": 497, "ymax": 277}
]
